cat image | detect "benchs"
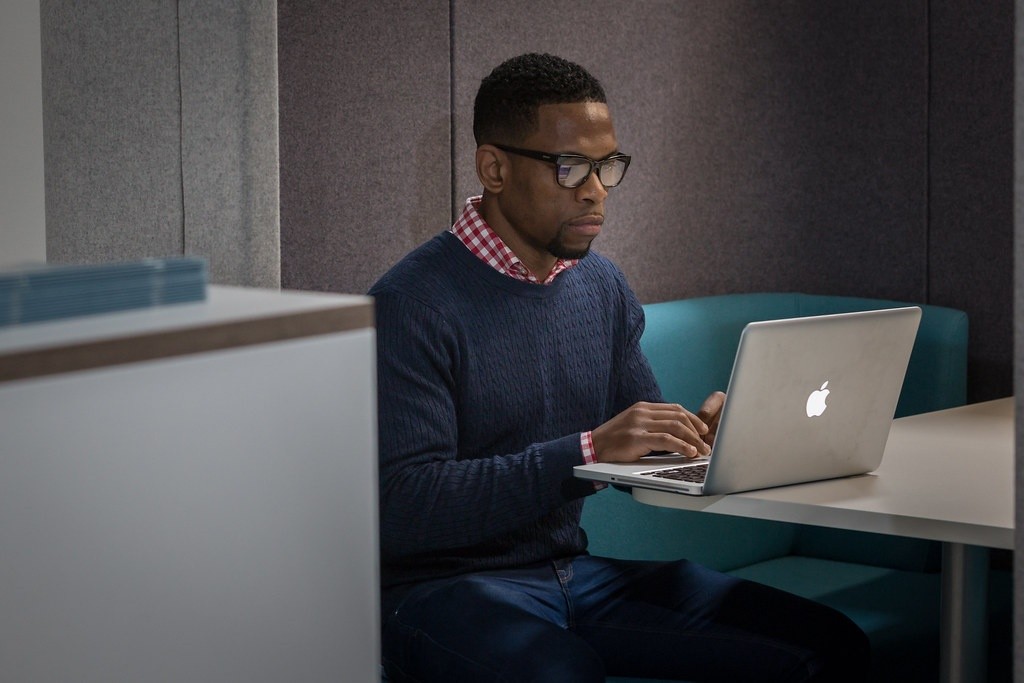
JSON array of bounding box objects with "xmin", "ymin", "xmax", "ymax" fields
[{"xmin": 580, "ymin": 292, "xmax": 1013, "ymax": 683}]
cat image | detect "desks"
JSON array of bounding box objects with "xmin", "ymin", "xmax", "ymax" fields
[{"xmin": 573, "ymin": 396, "xmax": 1015, "ymax": 683}]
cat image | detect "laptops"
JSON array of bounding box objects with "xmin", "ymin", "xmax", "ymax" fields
[{"xmin": 574, "ymin": 306, "xmax": 923, "ymax": 497}]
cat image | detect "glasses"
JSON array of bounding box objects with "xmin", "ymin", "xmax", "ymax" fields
[{"xmin": 486, "ymin": 142, "xmax": 632, "ymax": 189}]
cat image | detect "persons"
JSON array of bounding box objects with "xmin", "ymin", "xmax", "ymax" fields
[{"xmin": 365, "ymin": 51, "xmax": 879, "ymax": 683}]
